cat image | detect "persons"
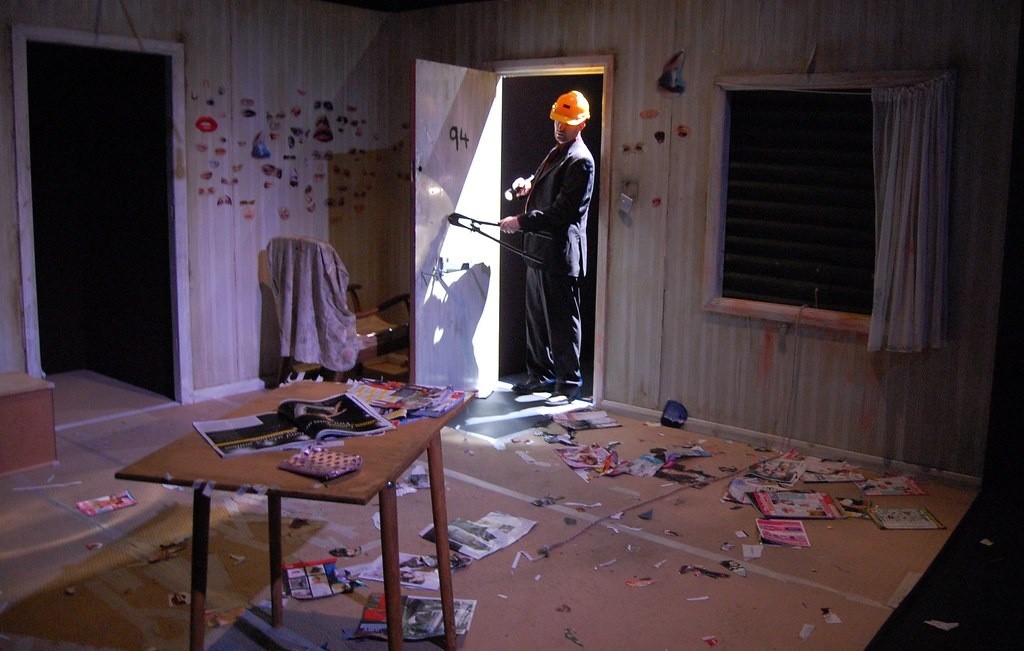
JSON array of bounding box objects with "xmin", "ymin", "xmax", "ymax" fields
[{"xmin": 498, "ymin": 90, "xmax": 595, "ymax": 406}]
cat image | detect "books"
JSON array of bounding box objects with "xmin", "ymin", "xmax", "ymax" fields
[
  {"xmin": 720, "ymin": 450, "xmax": 941, "ymax": 548},
  {"xmin": 193, "ymin": 392, "xmax": 394, "ymax": 460}
]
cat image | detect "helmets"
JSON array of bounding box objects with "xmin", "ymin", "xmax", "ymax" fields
[{"xmin": 550, "ymin": 91, "xmax": 590, "ymax": 126}]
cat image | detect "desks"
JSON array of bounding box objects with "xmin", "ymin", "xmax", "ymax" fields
[{"xmin": 114, "ymin": 377, "xmax": 475, "ymax": 650}]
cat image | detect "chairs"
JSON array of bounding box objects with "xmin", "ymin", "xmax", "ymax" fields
[{"xmin": 267, "ymin": 234, "xmax": 411, "ymax": 383}]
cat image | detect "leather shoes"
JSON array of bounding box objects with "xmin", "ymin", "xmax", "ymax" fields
[
  {"xmin": 546, "ymin": 387, "xmax": 582, "ymax": 405},
  {"xmin": 512, "ymin": 377, "xmax": 552, "ymax": 393}
]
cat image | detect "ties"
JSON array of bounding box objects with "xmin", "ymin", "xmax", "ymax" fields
[{"xmin": 542, "ymin": 149, "xmax": 560, "ymax": 173}]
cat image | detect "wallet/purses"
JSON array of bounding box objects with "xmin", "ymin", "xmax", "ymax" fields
[{"xmin": 277, "ymin": 444, "xmax": 362, "ymax": 478}]
